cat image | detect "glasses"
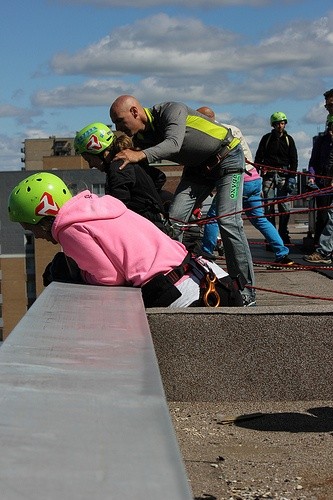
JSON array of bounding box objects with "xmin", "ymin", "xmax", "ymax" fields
[{"xmin": 324, "ymin": 104, "xmax": 333, "ymax": 108}]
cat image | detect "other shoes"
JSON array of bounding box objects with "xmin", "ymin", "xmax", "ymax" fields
[
  {"xmin": 270, "ymin": 256, "xmax": 294, "ymax": 266},
  {"xmin": 278, "ymin": 229, "xmax": 291, "ymax": 244},
  {"xmin": 239, "ymin": 287, "xmax": 256, "ymax": 307}
]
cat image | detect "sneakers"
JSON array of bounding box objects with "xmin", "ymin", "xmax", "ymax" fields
[{"xmin": 303, "ymin": 251, "xmax": 332, "ymax": 264}]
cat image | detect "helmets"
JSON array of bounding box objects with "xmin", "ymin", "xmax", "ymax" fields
[
  {"xmin": 270, "ymin": 111, "xmax": 288, "ymax": 126},
  {"xmin": 326, "ymin": 115, "xmax": 333, "ymax": 123},
  {"xmin": 74, "ymin": 123, "xmax": 115, "ymax": 154},
  {"xmin": 7, "ymin": 172, "xmax": 73, "ymax": 224}
]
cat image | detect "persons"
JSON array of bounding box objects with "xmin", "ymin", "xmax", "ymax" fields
[
  {"xmin": 7, "ymin": 171, "xmax": 249, "ymax": 308},
  {"xmin": 302, "ymin": 89, "xmax": 333, "ymax": 264},
  {"xmin": 195, "ymin": 106, "xmax": 296, "ymax": 268},
  {"xmin": 252, "ymin": 111, "xmax": 299, "ymax": 251},
  {"xmin": 308, "ymin": 112, "xmax": 333, "ymax": 249},
  {"xmin": 109, "ymin": 95, "xmax": 258, "ymax": 307},
  {"xmin": 73, "ymin": 122, "xmax": 177, "ymax": 240}
]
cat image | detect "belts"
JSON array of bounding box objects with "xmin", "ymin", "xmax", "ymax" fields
[{"xmin": 200, "ymin": 135, "xmax": 240, "ymax": 176}]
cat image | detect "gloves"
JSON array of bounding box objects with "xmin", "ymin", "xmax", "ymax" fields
[{"xmin": 286, "ymin": 178, "xmax": 296, "ymax": 194}]
